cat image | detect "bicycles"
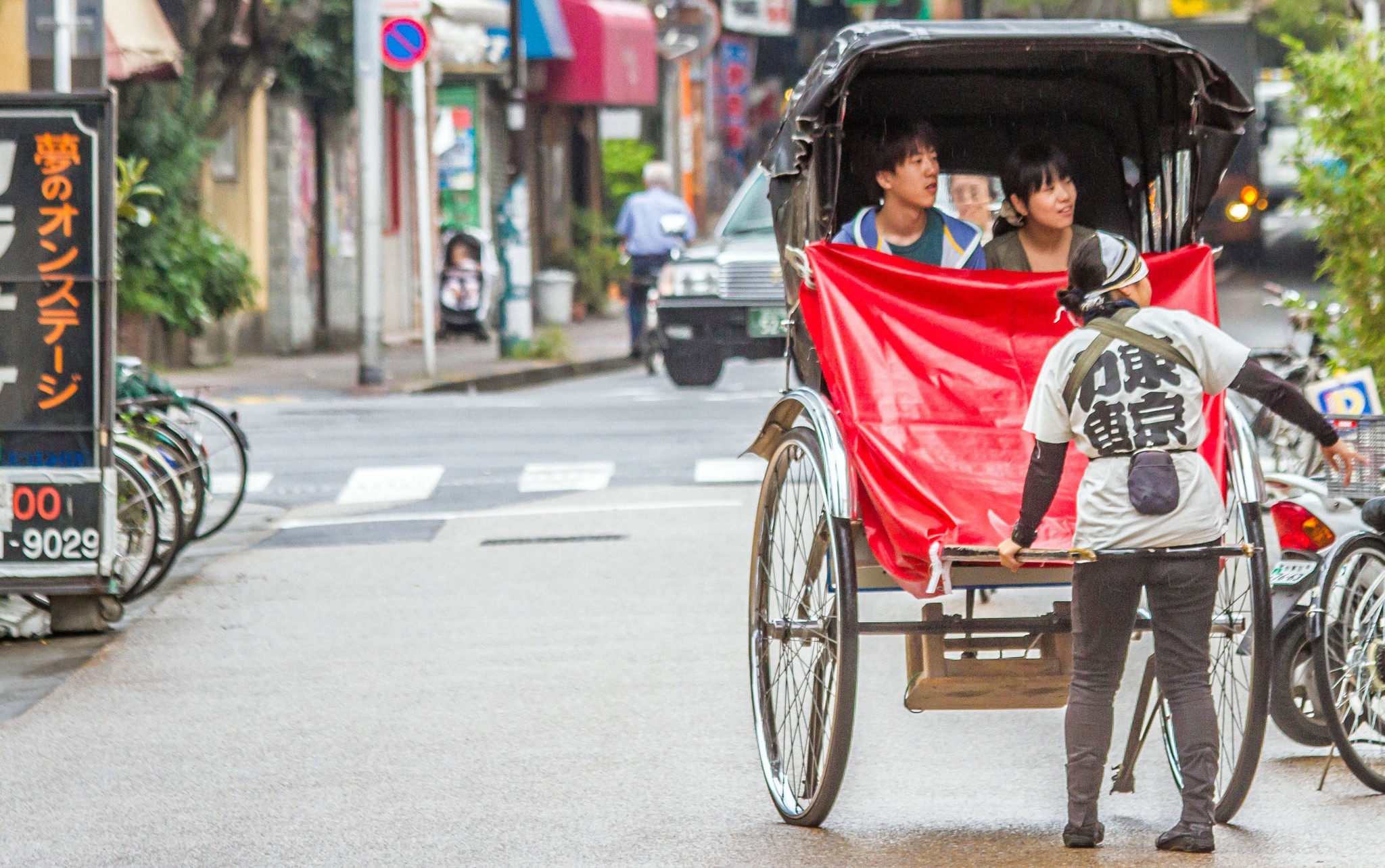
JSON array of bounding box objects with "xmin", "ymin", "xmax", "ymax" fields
[
  {"xmin": 17, "ymin": 356, "xmax": 250, "ymax": 614},
  {"xmin": 1242, "ymin": 312, "xmax": 1384, "ymax": 799}
]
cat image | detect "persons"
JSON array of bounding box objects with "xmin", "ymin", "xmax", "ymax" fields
[
  {"xmin": 996, "ymin": 230, "xmax": 1369, "ymax": 853},
  {"xmin": 983, "ymin": 142, "xmax": 1098, "ymax": 272},
  {"xmin": 947, "ymin": 175, "xmax": 996, "ymax": 232},
  {"xmin": 829, "ymin": 120, "xmax": 987, "ymax": 273},
  {"xmin": 615, "ymin": 159, "xmax": 698, "ymax": 361}
]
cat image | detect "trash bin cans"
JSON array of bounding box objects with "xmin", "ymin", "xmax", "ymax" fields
[{"xmin": 535, "ymin": 269, "xmax": 577, "ymax": 326}]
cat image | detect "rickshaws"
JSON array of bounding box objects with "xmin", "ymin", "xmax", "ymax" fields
[{"xmin": 734, "ymin": 18, "xmax": 1273, "ymax": 829}]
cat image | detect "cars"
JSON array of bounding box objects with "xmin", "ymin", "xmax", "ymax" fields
[{"xmin": 655, "ymin": 161, "xmax": 1006, "ymax": 388}]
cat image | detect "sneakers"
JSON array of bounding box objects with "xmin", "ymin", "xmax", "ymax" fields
[
  {"xmin": 1062, "ymin": 821, "xmax": 1105, "ymax": 848},
  {"xmin": 1155, "ymin": 820, "xmax": 1215, "ymax": 853}
]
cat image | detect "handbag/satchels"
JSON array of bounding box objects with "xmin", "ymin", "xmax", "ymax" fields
[{"xmin": 1128, "ymin": 451, "xmax": 1179, "ymax": 514}]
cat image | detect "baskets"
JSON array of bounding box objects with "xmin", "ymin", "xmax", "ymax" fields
[{"xmin": 1320, "ymin": 414, "xmax": 1385, "ymax": 498}]
